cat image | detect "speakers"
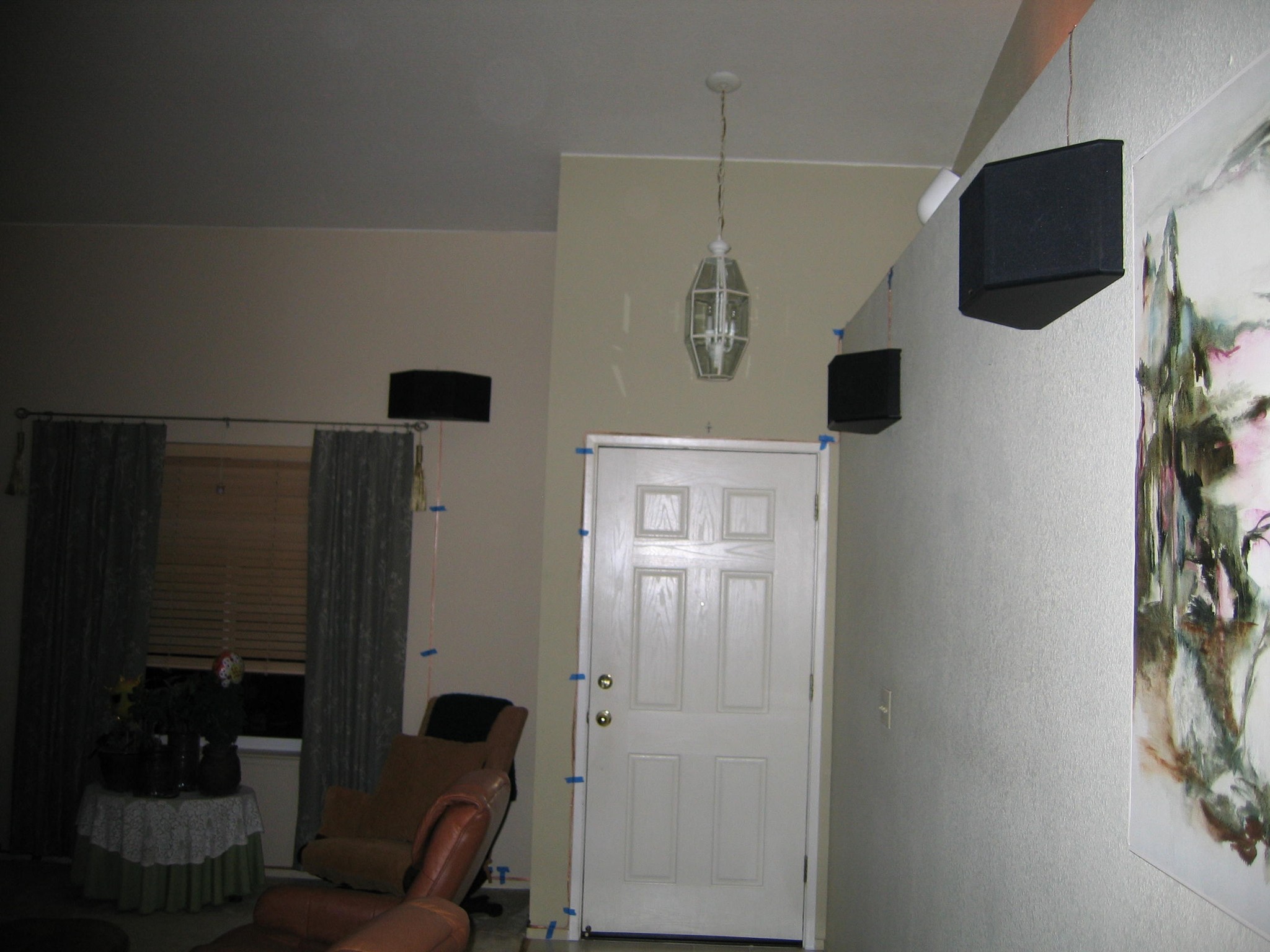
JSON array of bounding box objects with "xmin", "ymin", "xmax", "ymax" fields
[
  {"xmin": 388, "ymin": 370, "xmax": 490, "ymax": 420},
  {"xmin": 827, "ymin": 348, "xmax": 902, "ymax": 434},
  {"xmin": 960, "ymin": 140, "xmax": 1125, "ymax": 329}
]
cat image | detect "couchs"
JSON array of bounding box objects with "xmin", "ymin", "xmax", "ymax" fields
[
  {"xmin": 298, "ymin": 693, "xmax": 529, "ymax": 894},
  {"xmin": 192, "ymin": 768, "xmax": 512, "ymax": 952}
]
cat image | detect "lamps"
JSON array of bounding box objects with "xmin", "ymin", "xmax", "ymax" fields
[
  {"xmin": 388, "ymin": 368, "xmax": 492, "ymax": 424},
  {"xmin": 957, "ymin": 139, "xmax": 1125, "ymax": 330},
  {"xmin": 822, "ymin": 348, "xmax": 902, "ymax": 435},
  {"xmin": 684, "ymin": 72, "xmax": 753, "ymax": 383}
]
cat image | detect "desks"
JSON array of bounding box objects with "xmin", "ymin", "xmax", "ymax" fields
[{"xmin": 77, "ymin": 787, "xmax": 267, "ymax": 916}]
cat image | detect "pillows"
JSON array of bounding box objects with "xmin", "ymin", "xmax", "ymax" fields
[{"xmin": 362, "ymin": 731, "xmax": 483, "ymax": 841}]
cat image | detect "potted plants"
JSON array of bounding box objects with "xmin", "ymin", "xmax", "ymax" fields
[{"xmin": 92, "ymin": 671, "xmax": 248, "ymax": 796}]
